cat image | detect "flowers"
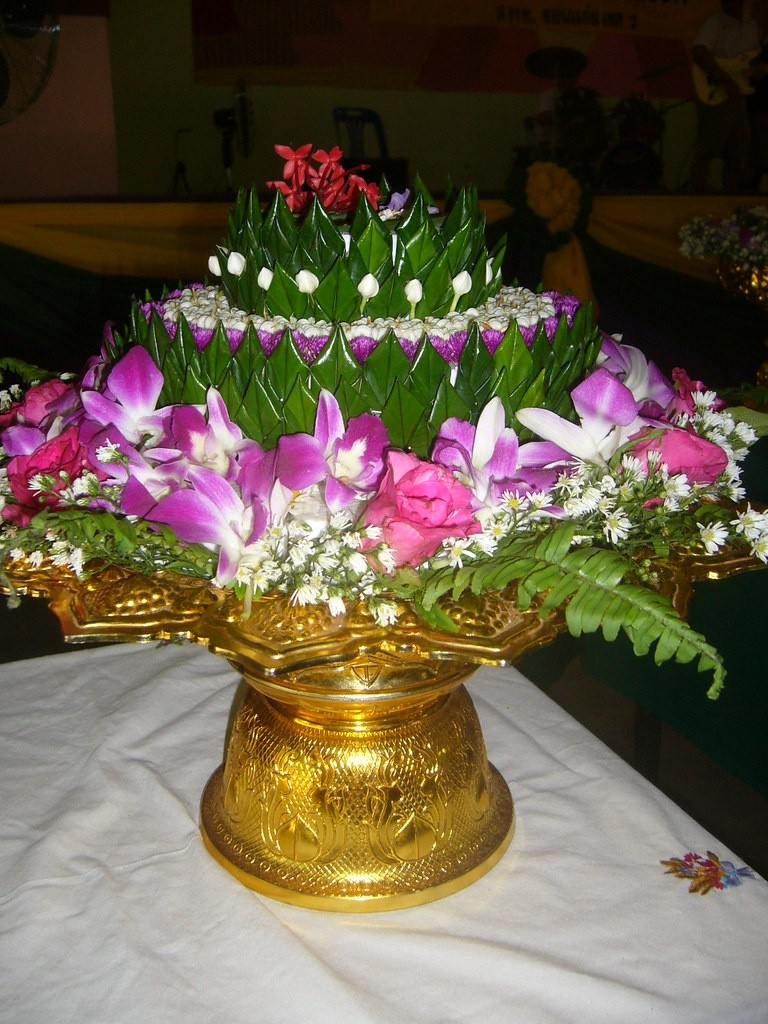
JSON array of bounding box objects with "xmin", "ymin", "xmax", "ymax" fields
[
  {"xmin": 608, "ymin": 91, "xmax": 665, "ymax": 138},
  {"xmin": 675, "ymin": 202, "xmax": 768, "ymax": 271},
  {"xmin": 558, "ymin": 86, "xmax": 609, "ymax": 128},
  {"xmin": 502, "ymin": 155, "xmax": 593, "ymax": 254},
  {"xmin": 0, "ymin": 142, "xmax": 768, "ymax": 702}
]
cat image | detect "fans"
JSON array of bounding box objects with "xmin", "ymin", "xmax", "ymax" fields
[
  {"xmin": 0, "ymin": 0, "xmax": 61, "ymax": 126},
  {"xmin": 212, "ymin": 74, "xmax": 257, "ymax": 194}
]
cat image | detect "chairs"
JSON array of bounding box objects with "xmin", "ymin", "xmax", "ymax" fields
[{"xmin": 333, "ymin": 105, "xmax": 410, "ymax": 187}]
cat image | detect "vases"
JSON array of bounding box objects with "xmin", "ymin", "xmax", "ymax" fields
[
  {"xmin": 0, "ymin": 542, "xmax": 768, "ymax": 913},
  {"xmin": 713, "ymin": 257, "xmax": 768, "ymax": 413}
]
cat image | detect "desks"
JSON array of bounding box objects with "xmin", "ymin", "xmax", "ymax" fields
[{"xmin": 0, "ymin": 638, "xmax": 768, "ymax": 1024}]
